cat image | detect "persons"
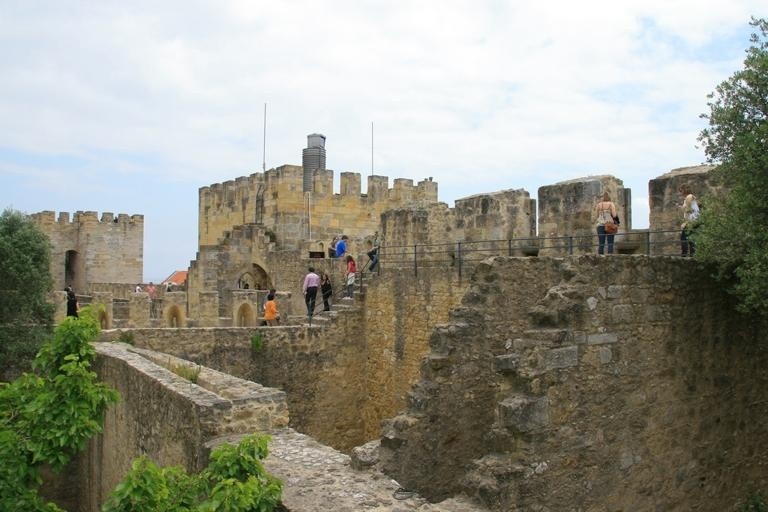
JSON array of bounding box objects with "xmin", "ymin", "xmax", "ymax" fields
[
  {"xmin": 334, "ymin": 235, "xmax": 351, "ymax": 257},
  {"xmin": 166, "ymin": 282, "xmax": 172, "ymax": 291},
  {"xmin": 327, "ymin": 236, "xmax": 339, "ymax": 258},
  {"xmin": 321, "ymin": 273, "xmax": 333, "ymax": 311},
  {"xmin": 592, "ymin": 191, "xmax": 621, "ymax": 254},
  {"xmin": 302, "ymin": 266, "xmax": 323, "ymax": 318},
  {"xmin": 67, "ymin": 292, "xmax": 80, "ymax": 319},
  {"xmin": 676, "ymin": 182, "xmax": 701, "ymax": 256},
  {"xmin": 264, "ymin": 294, "xmax": 280, "ymax": 326},
  {"xmin": 366, "ymin": 240, "xmax": 380, "ymax": 272},
  {"xmin": 67, "ymin": 284, "xmax": 72, "ymax": 291},
  {"xmin": 263, "ymin": 288, "xmax": 279, "ymax": 310},
  {"xmin": 135, "ymin": 282, "xmax": 142, "ymax": 292},
  {"xmin": 342, "ymin": 255, "xmax": 357, "ymax": 300},
  {"xmin": 144, "ymin": 282, "xmax": 157, "ymax": 299}
]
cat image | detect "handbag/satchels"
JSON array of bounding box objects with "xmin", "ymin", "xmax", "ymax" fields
[{"xmin": 603, "ymin": 221, "xmax": 617, "ymax": 233}]
